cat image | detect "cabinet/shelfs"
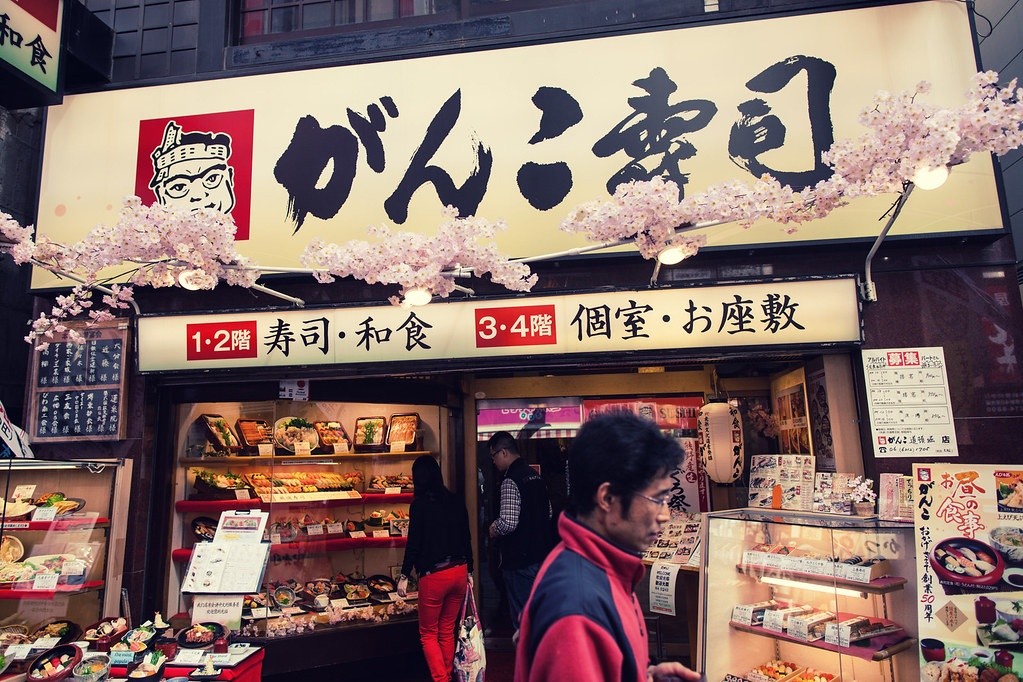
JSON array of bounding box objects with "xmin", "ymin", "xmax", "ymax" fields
[
  {"xmin": 729, "ymin": 563, "xmax": 917, "ymax": 662},
  {"xmin": 0, "ymin": 517, "xmax": 109, "ymax": 601},
  {"xmin": 171, "ymin": 451, "xmax": 441, "ymax": 567}
]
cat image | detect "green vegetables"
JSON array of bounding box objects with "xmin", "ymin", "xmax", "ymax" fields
[
  {"xmin": 284, "ymin": 417, "xmax": 313, "ymax": 430},
  {"xmin": 44, "ymin": 494, "xmax": 63, "ymax": 508},
  {"xmin": 134, "ymin": 626, "xmax": 151, "ymax": 632},
  {"xmin": 59, "ymin": 627, "xmax": 69, "ymax": 636},
  {"xmin": 207, "ymin": 625, "xmax": 215, "ymax": 631},
  {"xmin": 361, "ymin": 421, "xmax": 376, "ymax": 444},
  {"xmin": 150, "ymin": 649, "xmax": 164, "ymax": 666},
  {"xmin": 80, "ymin": 667, "xmax": 92, "ymax": 674},
  {"xmin": 0, "ymin": 654, "xmax": 5, "ymax": 670},
  {"xmin": 192, "ymin": 418, "xmax": 246, "ymax": 489}
]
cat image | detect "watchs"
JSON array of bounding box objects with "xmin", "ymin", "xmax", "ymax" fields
[{"xmin": 401, "ymin": 574, "xmax": 406, "ymax": 580}]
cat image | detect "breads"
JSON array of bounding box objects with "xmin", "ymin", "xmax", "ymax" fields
[{"xmin": 744, "ymin": 601, "xmax": 873, "ymax": 637}]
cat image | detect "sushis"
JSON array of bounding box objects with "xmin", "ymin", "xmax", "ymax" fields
[
  {"xmin": 935, "ymin": 544, "xmax": 996, "ymax": 576},
  {"xmin": 923, "ymin": 658, "xmax": 979, "ymax": 682},
  {"xmin": 248, "ymin": 473, "xmax": 414, "ymax": 494}
]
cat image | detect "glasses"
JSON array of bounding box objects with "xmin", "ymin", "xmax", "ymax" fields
[
  {"xmin": 633, "ymin": 491, "xmax": 670, "ymax": 512},
  {"xmin": 490, "ymin": 448, "xmax": 506, "ymax": 461}
]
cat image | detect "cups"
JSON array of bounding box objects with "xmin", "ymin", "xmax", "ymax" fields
[
  {"xmin": 971, "ymin": 650, "xmax": 992, "ymax": 666},
  {"xmin": 994, "ymin": 648, "xmax": 1014, "ymax": 669},
  {"xmin": 975, "ymin": 596, "xmax": 997, "ymax": 623},
  {"xmin": 920, "ymin": 638, "xmax": 945, "ymax": 662}
]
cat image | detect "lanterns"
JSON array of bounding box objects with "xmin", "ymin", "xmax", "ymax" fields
[{"xmin": 696, "ymin": 398, "xmax": 744, "ymax": 488}]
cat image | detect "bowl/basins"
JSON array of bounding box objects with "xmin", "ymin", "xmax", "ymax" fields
[
  {"xmin": 33, "ymin": 620, "xmax": 74, "ymax": 645},
  {"xmin": 1002, "ymin": 567, "xmax": 1023, "ymax": 587},
  {"xmin": 988, "ymin": 526, "xmax": 1023, "ymax": 566},
  {"xmin": 73, "ymin": 655, "xmax": 111, "ymax": 682},
  {"xmin": 127, "ymin": 627, "xmax": 156, "ymax": 644},
  {"xmin": 995, "ymin": 601, "xmax": 1023, "ymax": 621},
  {"xmin": 27, "ymin": 644, "xmax": 83, "ymax": 682},
  {"xmin": 929, "ymin": 536, "xmax": 1004, "ymax": 590},
  {"xmin": 109, "ymin": 641, "xmax": 149, "ymax": 656},
  {"xmin": 152, "ymin": 622, "xmax": 171, "ymax": 634}
]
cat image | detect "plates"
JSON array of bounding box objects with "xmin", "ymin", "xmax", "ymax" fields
[
  {"xmin": 187, "ymin": 667, "xmax": 222, "ymax": 680},
  {"xmin": 272, "ymin": 416, "xmax": 319, "ymax": 452},
  {"xmin": 0, "ymin": 562, "xmax": 49, "ymax": 583},
  {"xmin": 367, "ymin": 574, "xmax": 396, "ymax": 594},
  {"xmin": 191, "ymin": 516, "xmax": 218, "ymax": 543},
  {"xmin": 51, "ymin": 498, "xmax": 86, "ymax": 517},
  {"xmin": 34, "ymin": 492, "xmax": 65, "ymax": 507},
  {"xmin": 23, "ymin": 554, "xmax": 76, "ymax": 572},
  {"xmin": 273, "ymin": 586, "xmax": 296, "ymax": 607},
  {"xmin": 0, "ymin": 504, "xmax": 37, "ymax": 518},
  {"xmin": 177, "ymin": 622, "xmax": 224, "ymax": 648}
]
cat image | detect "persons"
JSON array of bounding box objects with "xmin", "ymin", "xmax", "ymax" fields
[
  {"xmin": 487, "ymin": 431, "xmax": 553, "ymax": 631},
  {"xmin": 514, "ymin": 413, "xmax": 700, "ymax": 682},
  {"xmin": 397, "ymin": 455, "xmax": 474, "ymax": 682}
]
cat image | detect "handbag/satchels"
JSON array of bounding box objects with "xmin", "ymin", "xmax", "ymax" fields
[{"xmin": 453, "ymin": 583, "xmax": 486, "ymax": 682}]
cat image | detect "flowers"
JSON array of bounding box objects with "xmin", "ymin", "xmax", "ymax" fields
[
  {"xmin": 0, "ymin": 209, "xmax": 537, "ymax": 360},
  {"xmin": 560, "ymin": 69, "xmax": 1023, "ymax": 274}
]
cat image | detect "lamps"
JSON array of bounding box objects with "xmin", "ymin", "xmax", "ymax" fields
[
  {"xmin": 404, "ymin": 283, "xmax": 474, "ymax": 306},
  {"xmin": 178, "ymin": 265, "xmax": 306, "ymax": 307},
  {"xmin": 859, "ymin": 161, "xmax": 949, "ymax": 303},
  {"xmin": 647, "ymin": 228, "xmax": 683, "ymax": 286}
]
